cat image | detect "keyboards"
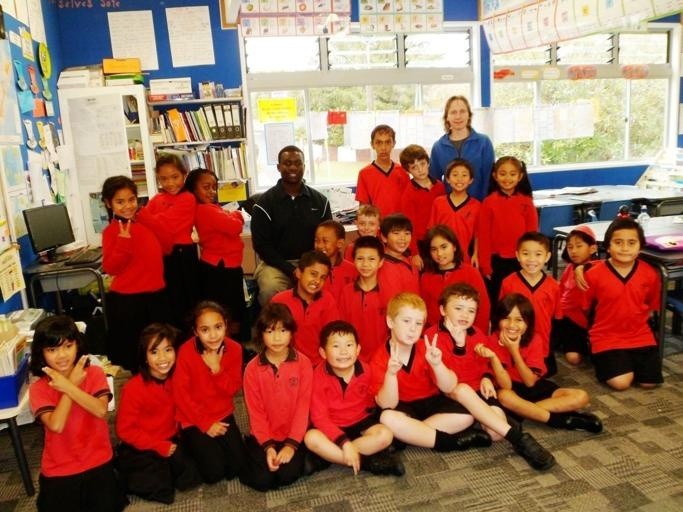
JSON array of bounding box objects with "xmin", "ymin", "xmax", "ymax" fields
[{"xmin": 64, "ymin": 246, "xmax": 103, "ymax": 265}]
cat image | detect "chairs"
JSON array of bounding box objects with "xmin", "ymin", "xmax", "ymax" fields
[
  {"xmin": 538, "ymin": 197, "xmax": 683, "ymax": 270},
  {"xmin": 651, "ymin": 289, "xmax": 683, "ymax": 339}
]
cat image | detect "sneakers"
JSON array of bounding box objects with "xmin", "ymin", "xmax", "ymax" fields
[
  {"xmin": 371, "ymin": 451, "xmax": 405, "ymax": 477},
  {"xmin": 573, "ymin": 413, "xmax": 603, "ymax": 433},
  {"xmin": 512, "ymin": 434, "xmax": 555, "ymax": 469},
  {"xmin": 455, "ymin": 430, "xmax": 492, "ymax": 450}
]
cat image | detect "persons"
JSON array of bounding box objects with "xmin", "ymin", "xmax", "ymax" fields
[
  {"xmin": 251, "ymin": 145, "xmax": 333, "ymax": 307},
  {"xmin": 560, "ymin": 217, "xmax": 664, "ymax": 390},
  {"xmin": 102, "ymin": 155, "xmax": 250, "ymax": 373},
  {"xmin": 241, "ymin": 203, "xmax": 604, "ymax": 493},
  {"xmin": 28, "ymin": 314, "xmax": 131, "ymax": 512},
  {"xmin": 429, "ymin": 96, "xmax": 494, "ymax": 204},
  {"xmin": 116, "ymin": 300, "xmax": 243, "ymax": 504},
  {"xmin": 355, "ymin": 126, "xmax": 539, "ymax": 335}
]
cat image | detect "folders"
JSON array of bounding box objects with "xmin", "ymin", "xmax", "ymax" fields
[{"xmin": 202, "ymin": 102, "xmax": 243, "ymax": 140}]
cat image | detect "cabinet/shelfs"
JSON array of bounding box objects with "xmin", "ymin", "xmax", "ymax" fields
[{"xmin": 56, "ymin": 83, "xmax": 254, "ymax": 250}]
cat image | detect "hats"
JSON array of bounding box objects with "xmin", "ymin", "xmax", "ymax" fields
[{"xmin": 561, "ymin": 227, "xmax": 595, "ymax": 261}]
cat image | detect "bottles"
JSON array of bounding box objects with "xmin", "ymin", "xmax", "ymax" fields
[{"xmin": 637, "ymin": 205, "xmax": 652, "ymax": 231}]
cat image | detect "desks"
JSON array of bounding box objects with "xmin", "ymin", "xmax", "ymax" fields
[
  {"xmin": 638, "ymin": 246, "xmax": 683, "ymax": 370},
  {"xmin": 533, "ymin": 184, "xmax": 683, "ymax": 225},
  {"xmin": 240, "ymin": 220, "xmax": 359, "ymax": 302},
  {"xmin": 0, "ymin": 321, "xmax": 87, "ymax": 497},
  {"xmin": 22, "ymin": 246, "xmax": 109, "ymax": 334}
]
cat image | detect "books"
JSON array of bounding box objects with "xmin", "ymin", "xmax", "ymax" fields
[
  {"xmin": 105, "ymin": 73, "xmax": 248, "ymax": 187},
  {"xmin": 0, "ymin": 220, "xmax": 50, "ymax": 379},
  {"xmin": 657, "ymin": 262, "xmax": 683, "ymax": 279}
]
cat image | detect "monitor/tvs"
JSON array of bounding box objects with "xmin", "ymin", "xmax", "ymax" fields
[{"xmin": 22, "ymin": 203, "xmax": 75, "ymax": 264}]
